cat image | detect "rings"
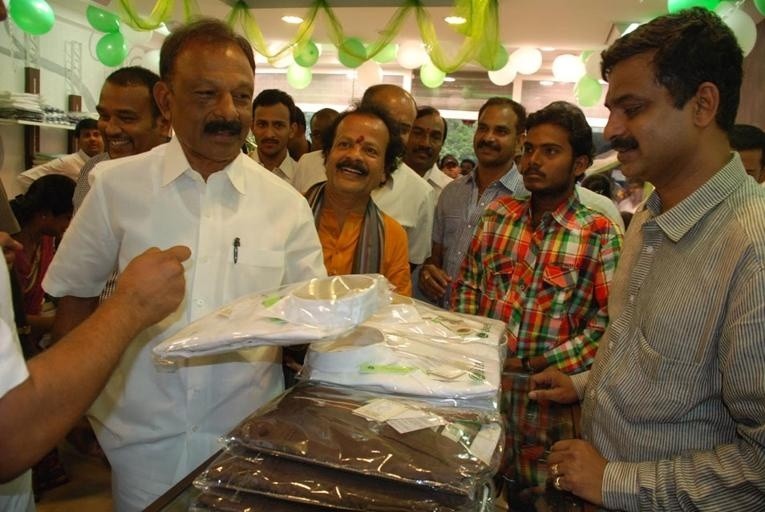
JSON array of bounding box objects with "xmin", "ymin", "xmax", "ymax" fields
[
  {"xmin": 551, "ymin": 464, "xmax": 558, "ymax": 477},
  {"xmin": 554, "ymin": 477, "xmax": 560, "ymax": 490}
]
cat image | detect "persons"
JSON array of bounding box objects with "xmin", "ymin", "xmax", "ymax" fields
[
  {"xmin": 530, "ymin": 6, "xmax": 765, "ymax": 512},
  {"xmin": 4, "ymin": 66, "xmax": 171, "ymax": 359},
  {"xmin": 244, "ymin": 85, "xmax": 644, "ymax": 343},
  {"xmin": 450, "ymin": 109, "xmax": 623, "ymax": 512},
  {"xmin": 0, "ymin": 245, "xmax": 190, "ymax": 512},
  {"xmin": 38, "ymin": 17, "xmax": 328, "ymax": 512},
  {"xmin": 729, "ymin": 124, "xmax": 765, "ymax": 183}
]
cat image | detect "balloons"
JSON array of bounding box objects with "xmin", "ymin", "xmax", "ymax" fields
[{"xmin": 10, "ymin": 0, "xmax": 764, "ymax": 106}]
